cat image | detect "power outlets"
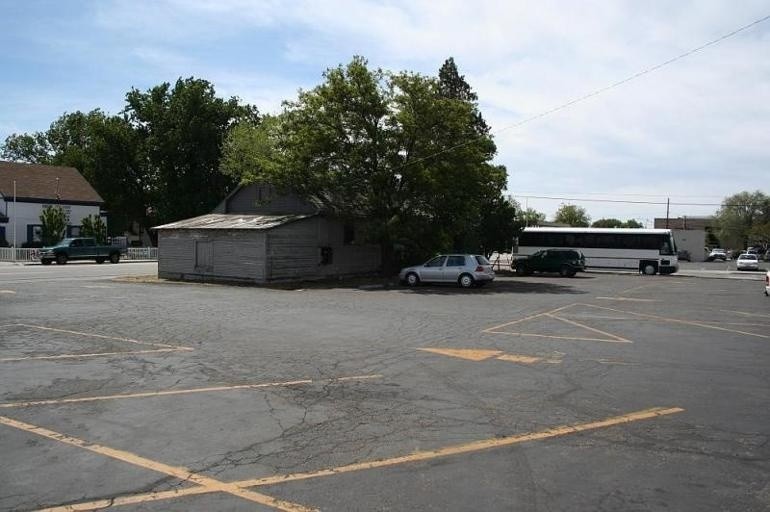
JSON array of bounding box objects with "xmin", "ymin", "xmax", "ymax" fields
[{"xmin": 39, "ymin": 236, "xmax": 123, "ymax": 265}]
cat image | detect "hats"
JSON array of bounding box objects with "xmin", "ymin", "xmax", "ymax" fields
[
  {"xmin": 708, "ymin": 247, "xmax": 727, "ymax": 262},
  {"xmin": 765, "ymin": 271, "xmax": 770, "ymax": 296},
  {"xmin": 511, "ymin": 249, "xmax": 585, "ymax": 277},
  {"xmin": 737, "ymin": 246, "xmax": 760, "ymax": 271}
]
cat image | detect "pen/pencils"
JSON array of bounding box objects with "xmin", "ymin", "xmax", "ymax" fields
[{"xmin": 399, "ymin": 254, "xmax": 496, "ymax": 288}]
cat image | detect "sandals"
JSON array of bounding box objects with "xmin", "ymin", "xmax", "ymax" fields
[{"xmin": 508, "ymin": 226, "xmax": 679, "ymax": 278}]
